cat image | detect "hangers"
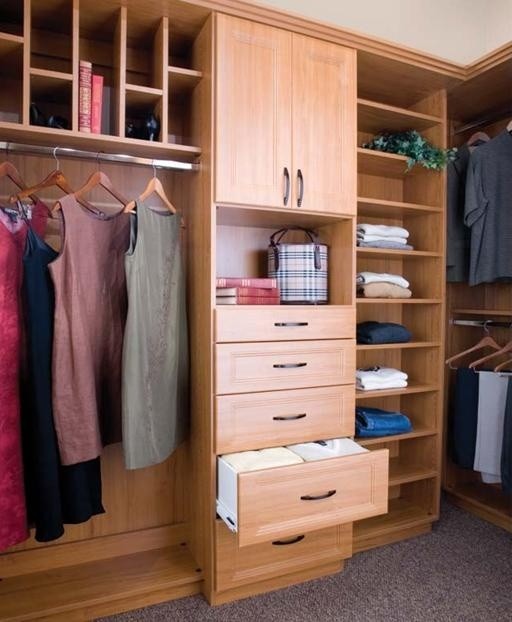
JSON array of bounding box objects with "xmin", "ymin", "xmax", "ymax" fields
[{"xmin": 0, "ymin": 140, "xmax": 186, "ymax": 227}]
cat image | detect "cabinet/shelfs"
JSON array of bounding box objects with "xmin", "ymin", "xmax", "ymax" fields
[{"xmin": 0, "ymin": 0, "xmax": 213, "ymax": 156}]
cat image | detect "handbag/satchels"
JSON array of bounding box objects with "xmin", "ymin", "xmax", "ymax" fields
[{"xmin": 267, "ymin": 225, "xmax": 329, "ymax": 305}]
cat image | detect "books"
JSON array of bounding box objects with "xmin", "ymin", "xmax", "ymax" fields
[
  {"xmin": 216, "ymin": 276, "xmax": 280, "ymax": 305},
  {"xmin": 78, "ymin": 57, "xmax": 112, "ymax": 136}
]
